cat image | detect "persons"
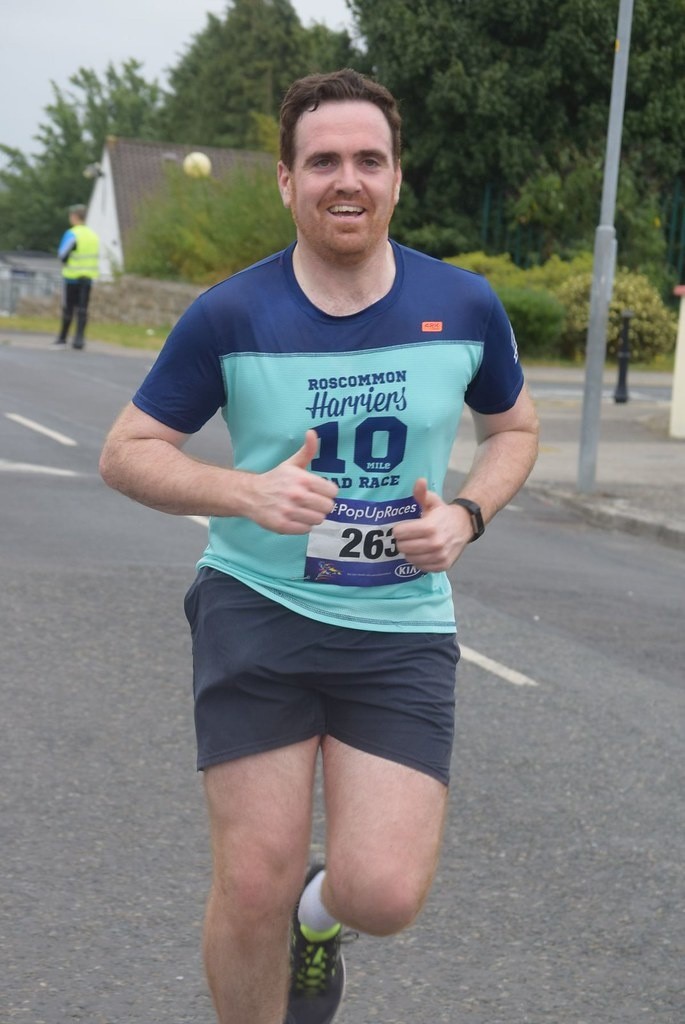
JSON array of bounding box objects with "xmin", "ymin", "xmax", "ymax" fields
[
  {"xmin": 54, "ymin": 203, "xmax": 98, "ymax": 346},
  {"xmin": 97, "ymin": 69, "xmax": 540, "ymax": 1023}
]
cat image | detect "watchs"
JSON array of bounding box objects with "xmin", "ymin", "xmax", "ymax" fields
[{"xmin": 449, "ymin": 498, "xmax": 485, "ymax": 543}]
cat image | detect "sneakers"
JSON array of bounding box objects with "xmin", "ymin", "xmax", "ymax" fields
[{"xmin": 283, "ymin": 865, "xmax": 345, "ymax": 1024}]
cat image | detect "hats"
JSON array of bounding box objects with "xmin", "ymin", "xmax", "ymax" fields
[{"xmin": 68, "ymin": 203, "xmax": 87, "ymax": 217}]
